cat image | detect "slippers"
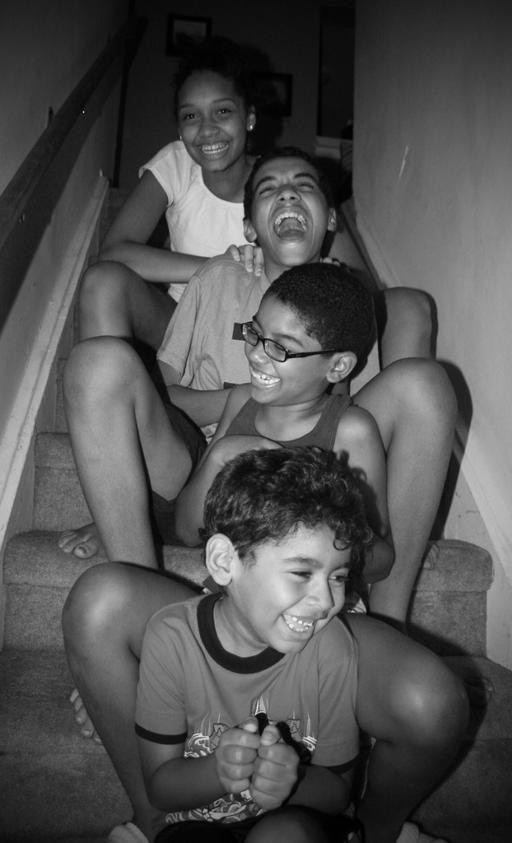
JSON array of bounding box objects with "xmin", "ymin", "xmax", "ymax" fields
[
  {"xmin": 346, "ymin": 818, "xmax": 444, "ymax": 843},
  {"xmin": 104, "ymin": 822, "xmax": 151, "ymax": 843}
]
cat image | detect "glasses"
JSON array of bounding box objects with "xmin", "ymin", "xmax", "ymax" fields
[{"xmin": 240, "ymin": 320, "xmax": 348, "ymax": 362}]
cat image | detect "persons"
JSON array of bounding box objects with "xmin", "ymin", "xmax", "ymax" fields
[
  {"xmin": 62, "ymin": 264, "xmax": 471, "ymax": 843},
  {"xmin": 62, "ymin": 147, "xmax": 458, "ymax": 745},
  {"xmin": 134, "ymin": 446, "xmax": 383, "ymax": 843},
  {"xmin": 56, "ymin": 57, "xmax": 439, "ymax": 570}
]
